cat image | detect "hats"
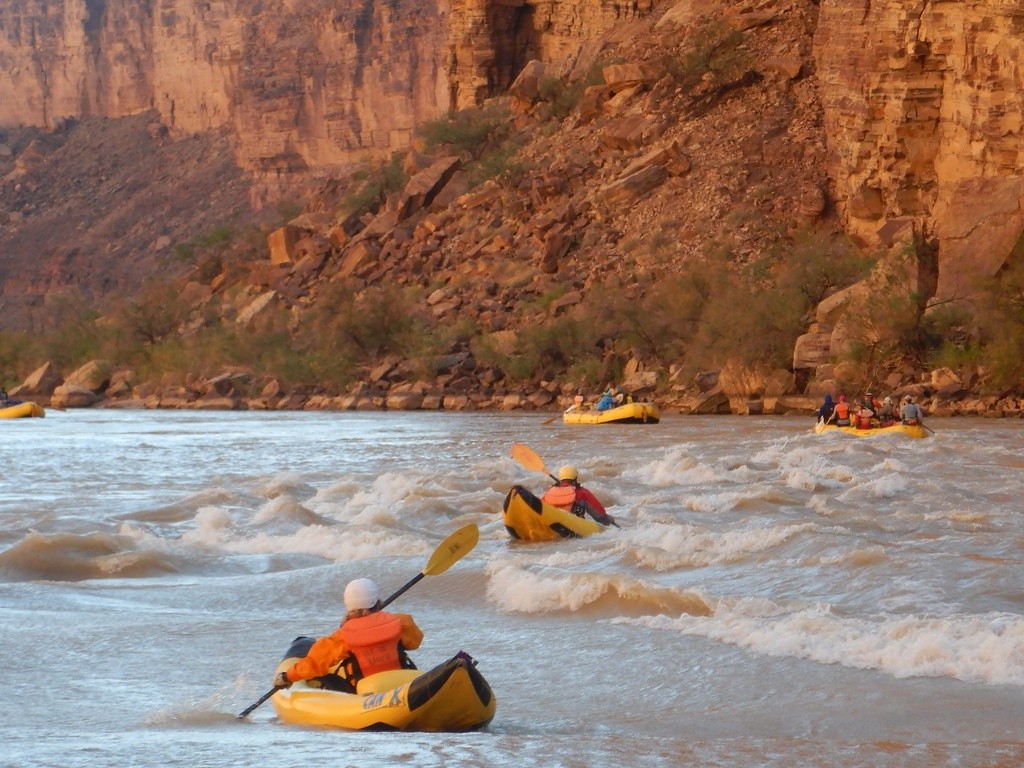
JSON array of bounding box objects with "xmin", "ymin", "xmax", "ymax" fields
[
  {"xmin": 839, "ymin": 395, "xmax": 846, "ymax": 401},
  {"xmin": 865, "ymin": 393, "xmax": 872, "ymax": 395},
  {"xmin": 884, "ymin": 397, "xmax": 891, "ymax": 403},
  {"xmin": 905, "ymin": 395, "xmax": 912, "ymax": 400}
]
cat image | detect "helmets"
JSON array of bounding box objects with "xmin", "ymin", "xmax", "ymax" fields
[
  {"xmin": 343, "ymin": 577, "xmax": 379, "ymax": 611},
  {"xmin": 559, "ymin": 464, "xmax": 578, "ymax": 480}
]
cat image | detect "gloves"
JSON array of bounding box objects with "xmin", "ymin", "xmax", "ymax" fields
[{"xmin": 273, "ymin": 672, "xmax": 293, "ymax": 691}]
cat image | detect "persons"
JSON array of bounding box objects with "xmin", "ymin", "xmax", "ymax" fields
[
  {"xmin": 542, "ymin": 465, "xmax": 615, "ymax": 526},
  {"xmin": 818, "ymin": 392, "xmax": 922, "ymax": 430},
  {"xmin": 598, "ymin": 381, "xmax": 627, "ymax": 411},
  {"xmin": 273, "ymin": 578, "xmax": 424, "ymax": 694},
  {"xmin": 0, "ymin": 387, "xmax": 8, "ymax": 400}
]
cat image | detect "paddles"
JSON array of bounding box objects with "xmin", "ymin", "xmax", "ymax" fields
[
  {"xmin": 543, "ymin": 393, "xmax": 602, "ymax": 424},
  {"xmin": 921, "ymin": 423, "xmax": 935, "ymax": 434},
  {"xmin": 510, "ymin": 442, "xmax": 621, "ymax": 527},
  {"xmin": 237, "ymin": 522, "xmax": 481, "ymax": 718},
  {"xmin": 817, "ymin": 419, "xmax": 831, "ymax": 434}
]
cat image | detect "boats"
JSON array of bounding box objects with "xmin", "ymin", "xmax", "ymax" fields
[
  {"xmin": 270, "ymin": 635, "xmax": 496, "ymax": 734},
  {"xmin": 814, "ymin": 418, "xmax": 931, "ymax": 438},
  {"xmin": 502, "ymin": 485, "xmax": 608, "ymax": 543},
  {"xmin": 563, "ymin": 402, "xmax": 661, "ymax": 425},
  {"xmin": 0, "ymin": 402, "xmax": 47, "ymax": 418}
]
